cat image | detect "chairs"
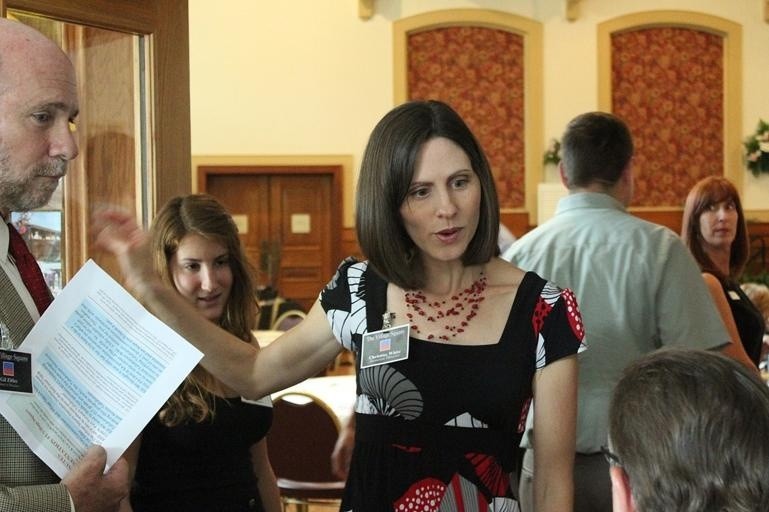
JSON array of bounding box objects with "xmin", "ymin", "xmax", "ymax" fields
[
  {"xmin": 272, "ymin": 309, "xmax": 307, "ymax": 332},
  {"xmin": 266, "ymin": 388, "xmax": 346, "ymax": 512}
]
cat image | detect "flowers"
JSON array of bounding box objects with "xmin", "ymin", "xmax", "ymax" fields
[{"xmin": 740, "ymin": 118, "xmax": 769, "ymax": 178}]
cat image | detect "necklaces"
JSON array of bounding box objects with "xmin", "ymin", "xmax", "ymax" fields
[{"xmin": 402, "ymin": 263, "xmax": 488, "ymax": 341}]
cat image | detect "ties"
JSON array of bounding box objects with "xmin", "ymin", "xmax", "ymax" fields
[{"xmin": 7, "ymin": 223, "xmax": 53, "ymax": 316}]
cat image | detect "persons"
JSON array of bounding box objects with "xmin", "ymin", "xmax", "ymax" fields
[
  {"xmin": 599, "ymin": 343, "xmax": 769, "ymax": 512},
  {"xmin": 0, "ymin": 15, "xmax": 131, "ymax": 511},
  {"xmin": 118, "ymin": 193, "xmax": 287, "ymax": 512},
  {"xmin": 86, "ymin": 98, "xmax": 590, "ymax": 512},
  {"xmin": 681, "ymin": 173, "xmax": 765, "ymax": 380},
  {"xmin": 329, "ymin": 218, "xmax": 520, "ymax": 480},
  {"xmin": 495, "ymin": 110, "xmax": 733, "ymax": 512}
]
